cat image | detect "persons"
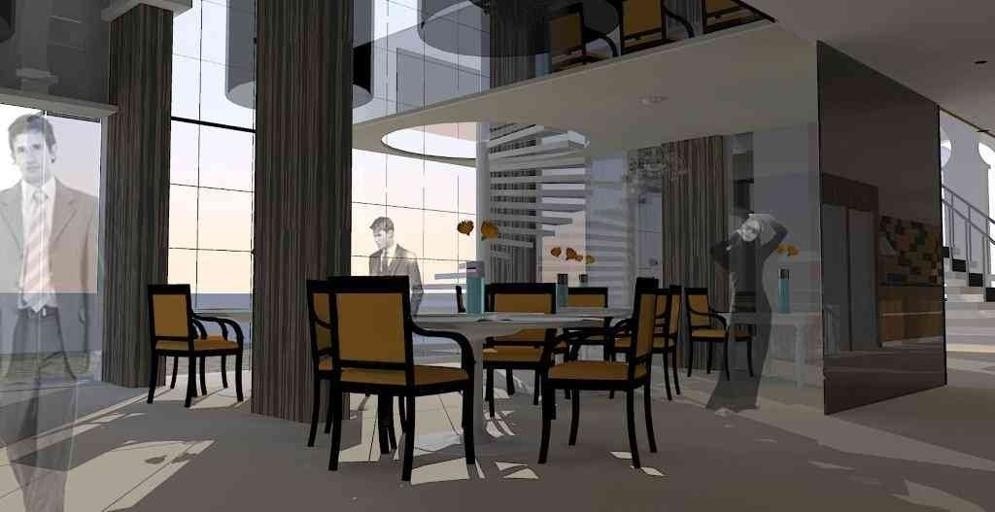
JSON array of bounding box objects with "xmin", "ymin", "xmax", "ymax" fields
[
  {"xmin": 0, "ymin": 114, "xmax": 102, "ymax": 512},
  {"xmin": 705, "ymin": 213, "xmax": 789, "ymax": 414},
  {"xmin": 368, "ymin": 215, "xmax": 424, "ymax": 319}
]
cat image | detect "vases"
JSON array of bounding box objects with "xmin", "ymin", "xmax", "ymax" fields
[
  {"xmin": 778, "ymin": 268, "xmax": 790, "ymax": 315},
  {"xmin": 578, "ymin": 274, "xmax": 588, "ymax": 286},
  {"xmin": 466, "ymin": 260, "xmax": 487, "ymax": 318},
  {"xmin": 556, "ymin": 273, "xmax": 569, "ymax": 307}
]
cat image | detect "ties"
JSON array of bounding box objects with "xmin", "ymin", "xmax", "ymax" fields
[
  {"xmin": 383, "ymin": 250, "xmax": 387, "ymax": 273},
  {"xmin": 23, "ymin": 188, "xmax": 54, "ymax": 313}
]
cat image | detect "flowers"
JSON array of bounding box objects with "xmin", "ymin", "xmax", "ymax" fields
[
  {"xmin": 775, "ymin": 242, "xmax": 800, "ymax": 257},
  {"xmin": 457, "ymin": 218, "xmax": 501, "ymax": 243},
  {"xmin": 576, "ymin": 255, "xmax": 596, "ymax": 266},
  {"xmin": 550, "ymin": 246, "xmax": 578, "ymax": 262}
]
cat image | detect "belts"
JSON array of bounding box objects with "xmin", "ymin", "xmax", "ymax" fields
[{"xmin": 18, "ymin": 305, "xmax": 58, "ymax": 318}]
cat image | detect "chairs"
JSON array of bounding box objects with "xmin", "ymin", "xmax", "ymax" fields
[
  {"xmin": 562, "ymin": 284, "xmax": 754, "ymax": 403},
  {"xmin": 306, "ymin": 277, "xmax": 660, "ymax": 487},
  {"xmin": 145, "ymin": 282, "xmax": 244, "ymax": 407}
]
